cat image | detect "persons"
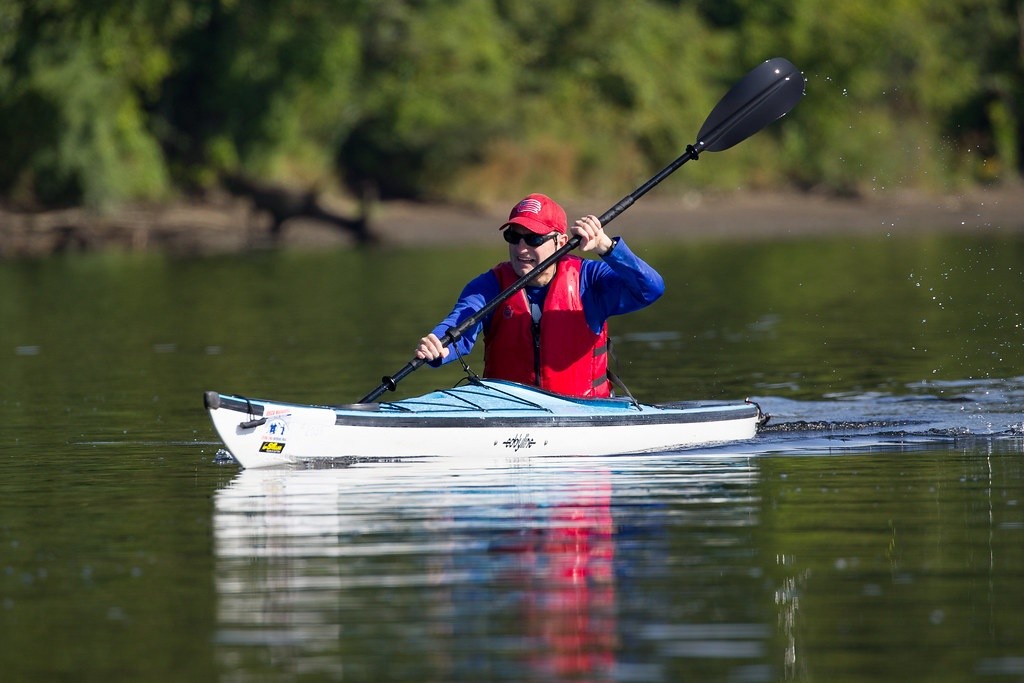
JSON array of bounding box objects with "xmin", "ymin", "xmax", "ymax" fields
[{"xmin": 414, "ymin": 192, "xmax": 666, "ymax": 398}]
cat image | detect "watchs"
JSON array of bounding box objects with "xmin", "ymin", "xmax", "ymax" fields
[{"xmin": 604, "ymin": 238, "xmax": 617, "ymax": 255}]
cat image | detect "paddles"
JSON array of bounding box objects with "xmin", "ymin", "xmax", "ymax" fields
[{"xmin": 358, "ymin": 58, "xmax": 807, "ymax": 407}]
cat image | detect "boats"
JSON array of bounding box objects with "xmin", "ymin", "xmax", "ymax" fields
[{"xmin": 198, "ymin": 377, "xmax": 769, "ymax": 471}]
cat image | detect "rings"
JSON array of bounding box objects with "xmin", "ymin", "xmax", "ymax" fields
[{"xmin": 581, "ymin": 217, "xmax": 588, "ymax": 222}]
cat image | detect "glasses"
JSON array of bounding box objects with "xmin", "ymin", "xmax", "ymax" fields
[{"xmin": 503, "ymin": 225, "xmax": 558, "ymax": 247}]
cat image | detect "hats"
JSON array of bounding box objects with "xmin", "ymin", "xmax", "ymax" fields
[{"xmin": 499, "ymin": 192, "xmax": 567, "ymax": 235}]
